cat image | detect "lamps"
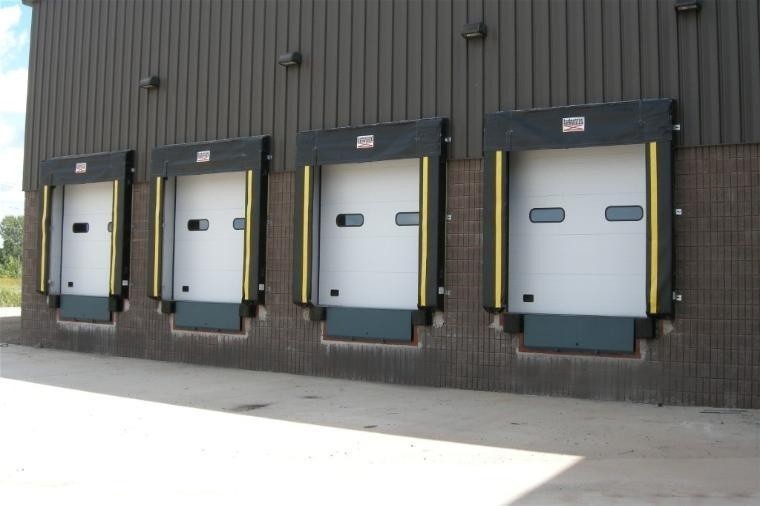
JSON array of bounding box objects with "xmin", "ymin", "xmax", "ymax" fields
[
  {"xmin": 139, "ymin": 75, "xmax": 162, "ymax": 87},
  {"xmin": 460, "ymin": 22, "xmax": 487, "ymax": 39},
  {"xmin": 278, "ymin": 51, "xmax": 300, "ymax": 65},
  {"xmin": 674, "ymin": 0, "xmax": 702, "ymax": 11}
]
